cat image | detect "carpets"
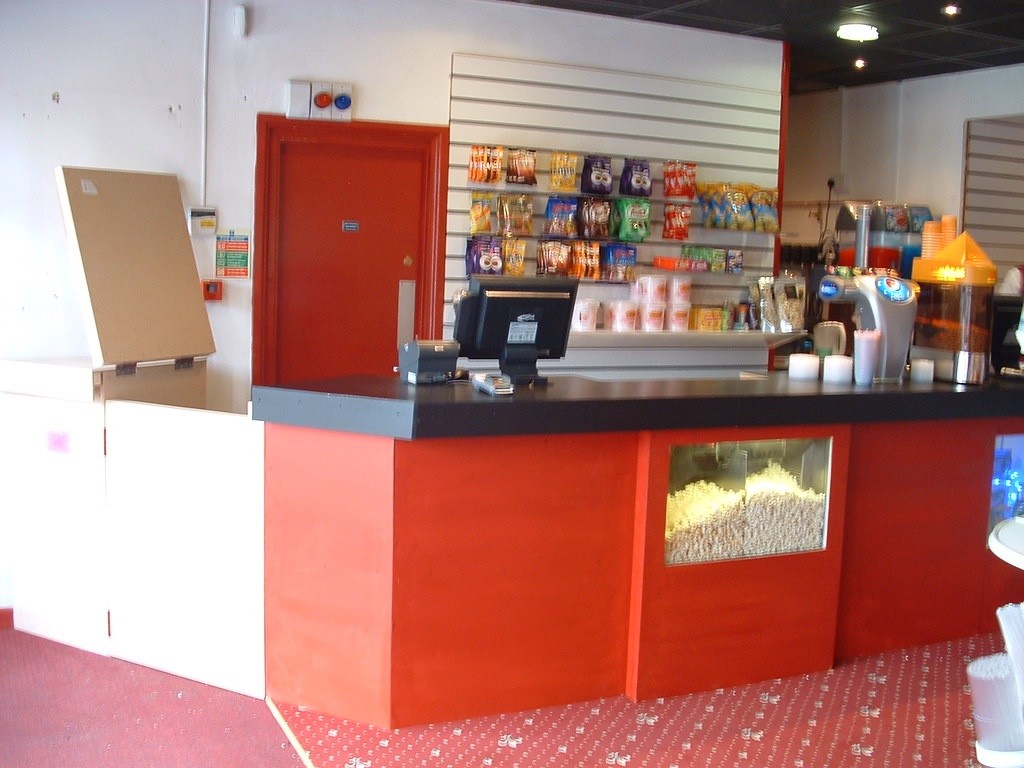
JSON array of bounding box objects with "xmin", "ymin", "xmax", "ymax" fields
[{"xmin": 268, "ymin": 626, "xmax": 1007, "ymax": 768}]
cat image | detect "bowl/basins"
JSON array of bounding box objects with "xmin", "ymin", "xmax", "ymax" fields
[{"xmin": 920, "ymin": 215, "xmax": 959, "ymax": 259}]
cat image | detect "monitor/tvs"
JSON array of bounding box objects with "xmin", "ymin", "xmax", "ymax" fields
[{"xmin": 453, "ymin": 275, "xmax": 578, "ymax": 387}]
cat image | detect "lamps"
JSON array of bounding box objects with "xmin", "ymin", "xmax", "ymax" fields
[{"xmin": 837, "ymin": 24, "xmax": 878, "ymax": 42}]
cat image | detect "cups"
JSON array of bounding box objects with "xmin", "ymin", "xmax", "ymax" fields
[
  {"xmin": 573, "ymin": 276, "xmax": 692, "ymax": 334},
  {"xmin": 854, "ymin": 329, "xmax": 881, "ymax": 386}
]
